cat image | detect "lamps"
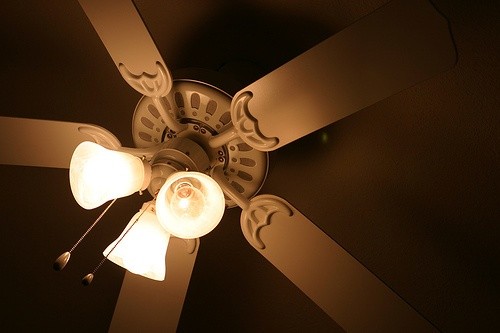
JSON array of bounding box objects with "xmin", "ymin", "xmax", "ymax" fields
[{"xmin": 69, "ymin": 141, "xmax": 226, "ymax": 282}]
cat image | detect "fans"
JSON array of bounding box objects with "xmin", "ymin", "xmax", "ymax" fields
[{"xmin": 0, "ymin": 0, "xmax": 500, "ymax": 333}]
